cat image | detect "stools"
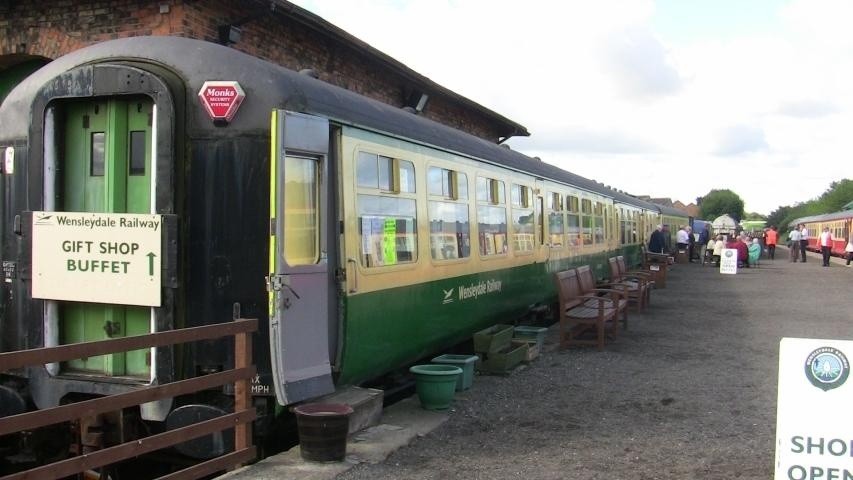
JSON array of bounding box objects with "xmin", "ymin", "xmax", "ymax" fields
[{"xmin": 703, "ymin": 249, "xmax": 760, "ymax": 269}]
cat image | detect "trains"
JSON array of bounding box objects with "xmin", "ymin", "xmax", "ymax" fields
[
  {"xmin": 691, "ymin": 215, "xmax": 769, "ymax": 261},
  {"xmin": 0, "ymin": 34, "xmax": 695, "ymax": 461},
  {"xmin": 786, "ymin": 209, "xmax": 852, "ymax": 261}
]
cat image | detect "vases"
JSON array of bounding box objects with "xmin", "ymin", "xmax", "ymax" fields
[{"xmin": 408, "ymin": 323, "xmax": 549, "ymax": 411}]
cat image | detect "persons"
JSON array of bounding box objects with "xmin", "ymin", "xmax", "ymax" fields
[
  {"xmin": 817, "ymin": 226, "xmax": 836, "ymax": 267},
  {"xmin": 648, "ymin": 223, "xmax": 778, "ymax": 268},
  {"xmin": 788, "ymin": 227, "xmax": 801, "ymax": 262},
  {"xmin": 843, "ymin": 231, "xmax": 853, "ymax": 265},
  {"xmin": 798, "ymin": 224, "xmax": 809, "ymax": 262}
]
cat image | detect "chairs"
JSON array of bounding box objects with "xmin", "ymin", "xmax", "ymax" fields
[{"xmin": 554, "ymin": 239, "xmax": 669, "ymax": 352}]
